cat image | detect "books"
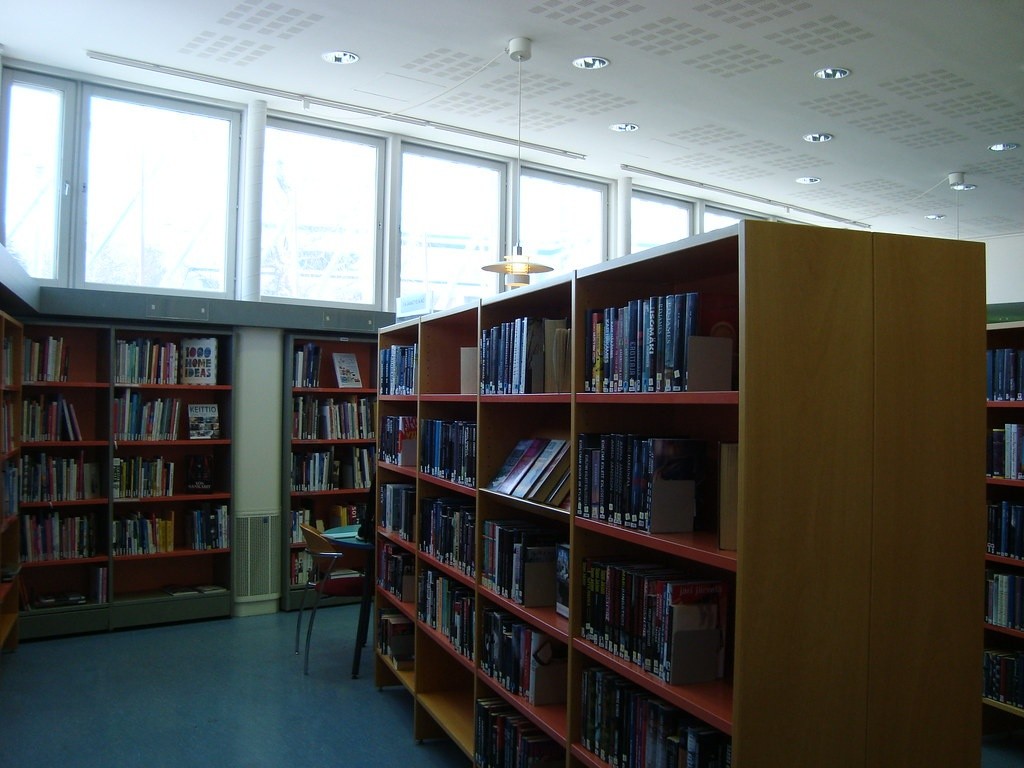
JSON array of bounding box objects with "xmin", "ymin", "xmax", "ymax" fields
[
  {"xmin": 0, "ymin": 284, "xmax": 738, "ymax": 767},
  {"xmin": 983, "ymin": 339, "xmax": 1024, "ymax": 708}
]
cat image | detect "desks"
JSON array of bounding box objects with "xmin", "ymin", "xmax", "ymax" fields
[{"xmin": 321, "ymin": 525, "xmax": 376, "ymax": 680}]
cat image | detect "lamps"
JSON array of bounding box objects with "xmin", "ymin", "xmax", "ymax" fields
[{"xmin": 481, "ymin": 37, "xmax": 555, "ymax": 287}]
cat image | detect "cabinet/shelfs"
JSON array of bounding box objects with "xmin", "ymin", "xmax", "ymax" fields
[
  {"xmin": 985, "ymin": 302, "xmax": 1024, "ymax": 718},
  {"xmin": 19, "ymin": 319, "xmax": 235, "ymax": 640},
  {"xmin": 870, "ymin": 231, "xmax": 988, "ymax": 768},
  {"xmin": 0, "ymin": 310, "xmax": 24, "ymax": 649},
  {"xmin": 281, "ymin": 334, "xmax": 378, "ymax": 612},
  {"xmin": 377, "ymin": 217, "xmax": 871, "ymax": 768}
]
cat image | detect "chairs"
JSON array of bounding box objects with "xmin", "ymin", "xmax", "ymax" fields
[{"xmin": 294, "ymin": 523, "xmax": 375, "ymax": 675}]
